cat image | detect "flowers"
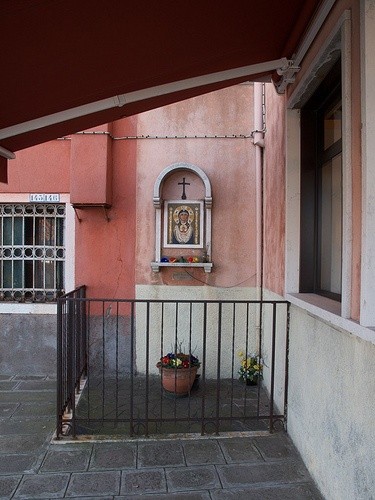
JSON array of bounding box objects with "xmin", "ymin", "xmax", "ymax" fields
[
  {"xmin": 238, "ymin": 351, "xmax": 263, "ymax": 383},
  {"xmin": 157, "ymin": 353, "xmax": 201, "ymax": 368}
]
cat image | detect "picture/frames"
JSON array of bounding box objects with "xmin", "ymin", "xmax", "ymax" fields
[{"xmin": 164, "ymin": 199, "xmax": 204, "ymax": 248}]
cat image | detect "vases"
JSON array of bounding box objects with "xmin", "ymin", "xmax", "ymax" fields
[
  {"xmin": 246, "ymin": 379, "xmax": 258, "ymax": 386},
  {"xmin": 160, "ymin": 368, "xmax": 198, "ymax": 394}
]
